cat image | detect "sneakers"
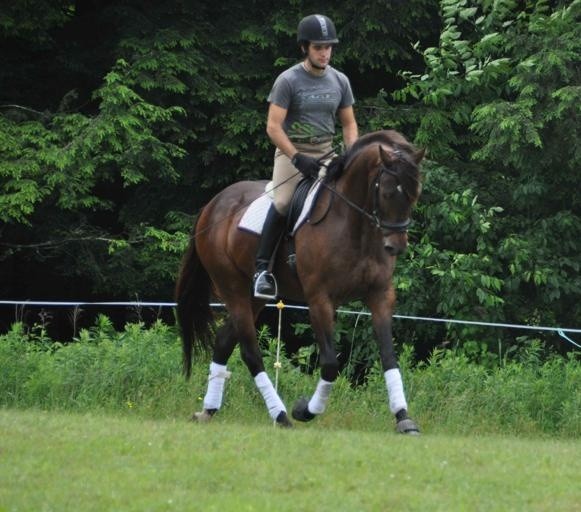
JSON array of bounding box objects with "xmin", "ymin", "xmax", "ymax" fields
[{"xmin": 251, "ymin": 273, "xmax": 275, "ymax": 295}]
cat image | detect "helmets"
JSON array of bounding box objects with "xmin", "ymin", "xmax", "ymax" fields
[{"xmin": 296, "ymin": 14, "xmax": 340, "ymax": 48}]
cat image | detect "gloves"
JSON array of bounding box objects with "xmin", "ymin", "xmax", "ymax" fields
[{"xmin": 292, "ymin": 152, "xmax": 320, "ymax": 180}]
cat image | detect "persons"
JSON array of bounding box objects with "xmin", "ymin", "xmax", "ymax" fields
[{"xmin": 251, "ymin": 14, "xmax": 361, "ymax": 301}]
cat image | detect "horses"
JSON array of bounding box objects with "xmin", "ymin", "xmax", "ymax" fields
[{"xmin": 175, "ymin": 130, "xmax": 427, "ymax": 435}]
